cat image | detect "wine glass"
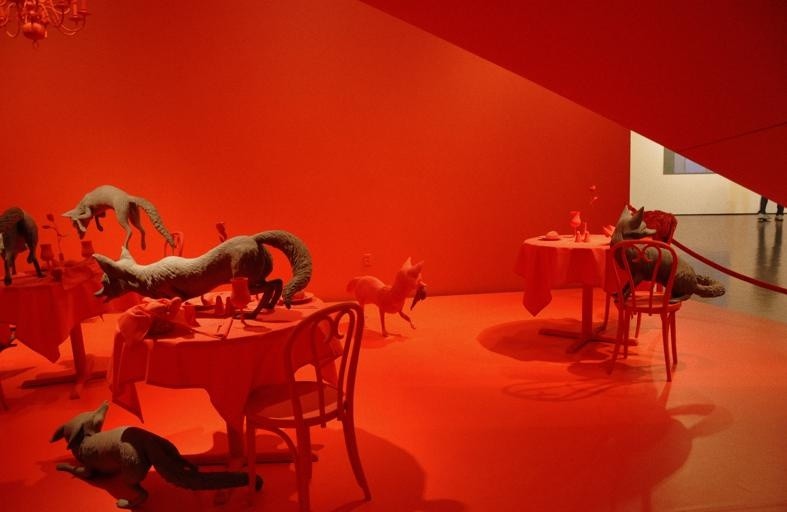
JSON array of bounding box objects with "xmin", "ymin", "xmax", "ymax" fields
[
  {"xmin": 231, "ymin": 277, "xmax": 251, "ymax": 330},
  {"xmin": 81, "ymin": 240, "xmax": 95, "ymax": 263},
  {"xmin": 39, "ymin": 244, "xmax": 54, "ymax": 271},
  {"xmin": 569, "ymin": 211, "xmax": 581, "ymax": 242}
]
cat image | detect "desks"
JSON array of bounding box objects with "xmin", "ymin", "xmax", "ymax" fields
[
  {"xmin": 521, "ymin": 231, "xmax": 653, "ymax": 354},
  {"xmin": 1, "ymin": 261, "xmax": 144, "ymax": 400},
  {"xmin": 108, "ymin": 290, "xmax": 346, "ymax": 463}
]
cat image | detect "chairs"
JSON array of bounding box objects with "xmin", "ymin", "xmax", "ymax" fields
[
  {"xmin": 602, "ymin": 208, "xmax": 679, "ymax": 339},
  {"xmin": 606, "ymin": 241, "xmax": 682, "ymax": 382},
  {"xmin": 243, "ymin": 300, "xmax": 371, "ymax": 512},
  {"xmin": 163, "ymin": 230, "xmax": 182, "ymax": 257}
]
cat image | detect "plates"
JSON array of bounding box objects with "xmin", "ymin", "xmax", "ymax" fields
[
  {"xmin": 537, "ymin": 235, "xmax": 562, "ymax": 240},
  {"xmin": 280, "ymin": 290, "xmax": 313, "ymax": 303}
]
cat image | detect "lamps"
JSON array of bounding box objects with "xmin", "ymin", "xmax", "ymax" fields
[{"xmin": 0, "ymin": 0, "xmax": 92, "ymax": 49}]
